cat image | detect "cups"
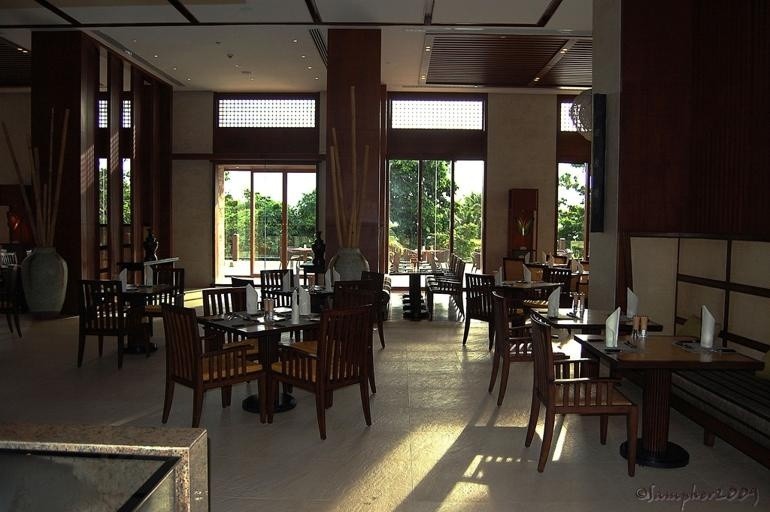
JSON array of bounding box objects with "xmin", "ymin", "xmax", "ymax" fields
[
  {"xmin": 606, "ymin": 329, "xmax": 618, "ymax": 347},
  {"xmin": 292, "ymin": 305, "xmax": 299, "ymax": 324},
  {"xmin": 548, "ymin": 303, "xmax": 558, "ymax": 318},
  {"xmin": 700, "ymin": 328, "xmax": 714, "ymax": 348}
]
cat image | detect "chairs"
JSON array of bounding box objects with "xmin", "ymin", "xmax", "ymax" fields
[{"xmin": 1, "ymin": 265, "xmax": 24, "ymax": 340}]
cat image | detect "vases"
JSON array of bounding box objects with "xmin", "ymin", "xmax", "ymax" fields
[{"xmin": 21, "ymin": 247, "xmax": 68, "ymax": 314}]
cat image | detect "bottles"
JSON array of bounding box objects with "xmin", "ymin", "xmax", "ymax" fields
[
  {"xmin": 143, "ymin": 228, "xmax": 159, "ymax": 261},
  {"xmin": 573, "ymin": 292, "xmax": 585, "ymax": 311},
  {"xmin": 311, "ymin": 231, "xmax": 325, "ymax": 265},
  {"xmin": 640, "ymin": 312, "xmax": 648, "ymax": 337},
  {"xmin": 264, "ymin": 298, "xmax": 275, "ymax": 319},
  {"xmin": 631, "ymin": 313, "xmax": 640, "ymax": 338}
]
